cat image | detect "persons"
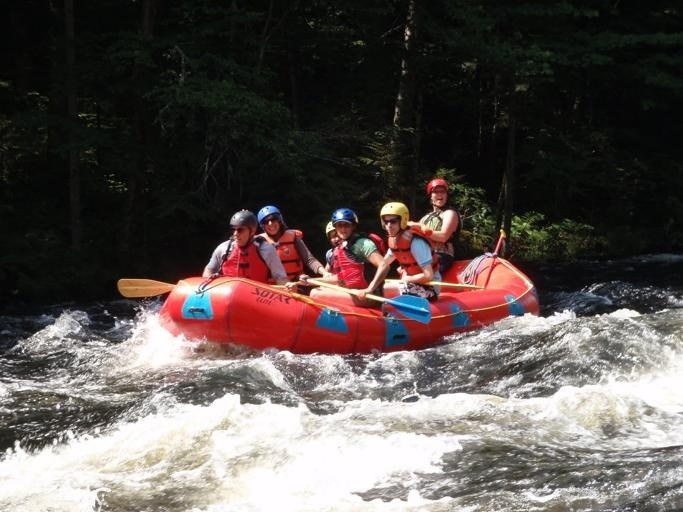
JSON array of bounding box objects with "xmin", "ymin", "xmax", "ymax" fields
[
  {"xmin": 321, "ymin": 221, "xmax": 344, "ymax": 279},
  {"xmin": 297, "ymin": 208, "xmax": 390, "ymax": 308},
  {"xmin": 252, "ymin": 204, "xmax": 335, "ymax": 286},
  {"xmin": 201, "ymin": 208, "xmax": 297, "ymax": 296},
  {"xmin": 377, "ymin": 202, "xmax": 443, "ymax": 302},
  {"xmin": 414, "ymin": 178, "xmax": 462, "ymax": 279}
]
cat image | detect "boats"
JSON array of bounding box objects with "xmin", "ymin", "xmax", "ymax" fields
[{"xmin": 153, "ymin": 253, "xmax": 541, "ymax": 357}]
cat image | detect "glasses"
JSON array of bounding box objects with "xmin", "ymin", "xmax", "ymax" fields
[
  {"xmin": 432, "ymin": 189, "xmax": 446, "ymax": 194},
  {"xmin": 382, "ymin": 217, "xmax": 399, "ymax": 224},
  {"xmin": 336, "ymin": 223, "xmax": 350, "ymax": 228},
  {"xmin": 230, "ymin": 228, "xmax": 244, "ymax": 233},
  {"xmin": 262, "ymin": 216, "xmax": 277, "ymax": 224}
]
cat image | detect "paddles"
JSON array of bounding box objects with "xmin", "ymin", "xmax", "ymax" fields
[
  {"xmin": 382, "ymin": 277, "xmax": 485, "ymax": 288},
  {"xmin": 305, "ymin": 278, "xmax": 430, "ymax": 324},
  {"xmin": 118, "ymin": 279, "xmax": 176, "ymax": 297}
]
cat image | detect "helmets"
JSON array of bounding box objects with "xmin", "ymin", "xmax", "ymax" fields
[
  {"xmin": 257, "ymin": 206, "xmax": 280, "ymax": 224},
  {"xmin": 331, "ymin": 208, "xmax": 358, "ymax": 227},
  {"xmin": 426, "ymin": 178, "xmax": 448, "ymax": 195},
  {"xmin": 229, "ymin": 210, "xmax": 257, "ymax": 235},
  {"xmin": 379, "ymin": 202, "xmax": 409, "ymax": 230},
  {"xmin": 325, "ymin": 220, "xmax": 335, "ymax": 239}
]
